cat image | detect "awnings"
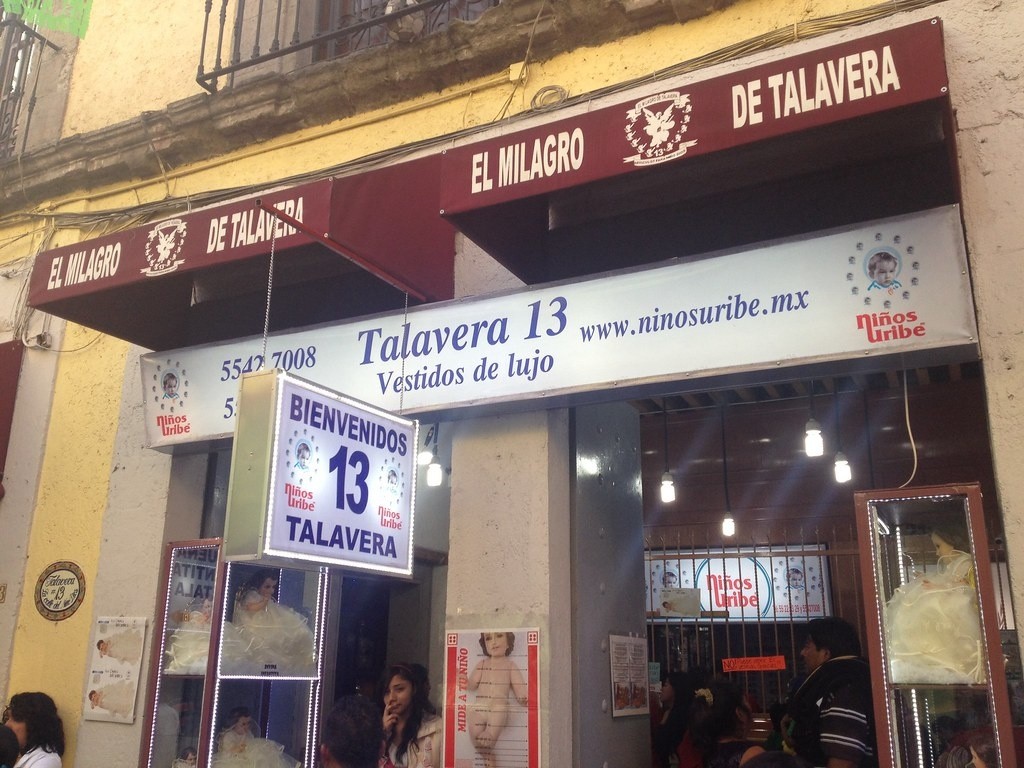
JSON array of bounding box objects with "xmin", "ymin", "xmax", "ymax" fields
[
  {"xmin": 437, "ymin": 18, "xmax": 965, "ymax": 287},
  {"xmin": 24, "ymin": 150, "xmax": 457, "ymax": 351}
]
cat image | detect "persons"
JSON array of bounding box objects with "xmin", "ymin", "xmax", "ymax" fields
[
  {"xmin": 222, "ymin": 568, "xmax": 315, "ymax": 665},
  {"xmin": 212, "ymin": 708, "xmax": 302, "ymax": 768},
  {"xmin": 656, "ymin": 618, "xmax": 879, "ymax": 768},
  {"xmin": 882, "ymin": 520, "xmax": 985, "ymax": 686},
  {"xmin": 456, "ymin": 633, "xmax": 527, "ymax": 768},
  {"xmin": 166, "ymin": 598, "xmax": 213, "ymax": 677},
  {"xmin": 929, "ymin": 716, "xmax": 999, "ymax": 768},
  {"xmin": 170, "ymin": 746, "xmax": 198, "ymax": 768},
  {"xmin": 1, "ymin": 692, "xmax": 65, "ymax": 768},
  {"xmin": 318, "ymin": 660, "xmax": 442, "ymax": 768}
]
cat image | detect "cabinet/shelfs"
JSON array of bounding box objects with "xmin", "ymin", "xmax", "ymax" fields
[
  {"xmin": 138, "ymin": 539, "xmax": 330, "ymax": 768},
  {"xmin": 854, "ymin": 482, "xmax": 1017, "ymax": 768}
]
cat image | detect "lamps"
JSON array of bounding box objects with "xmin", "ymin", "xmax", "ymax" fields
[
  {"xmin": 427, "ymin": 423, "xmax": 442, "ymax": 487},
  {"xmin": 720, "ymin": 390, "xmax": 735, "ymax": 536},
  {"xmin": 417, "ymin": 423, "xmax": 434, "ymax": 465},
  {"xmin": 660, "ymin": 397, "xmax": 675, "ymax": 502},
  {"xmin": 805, "ymin": 379, "xmax": 824, "ymax": 457},
  {"xmin": 834, "ymin": 377, "xmax": 852, "ymax": 483}
]
